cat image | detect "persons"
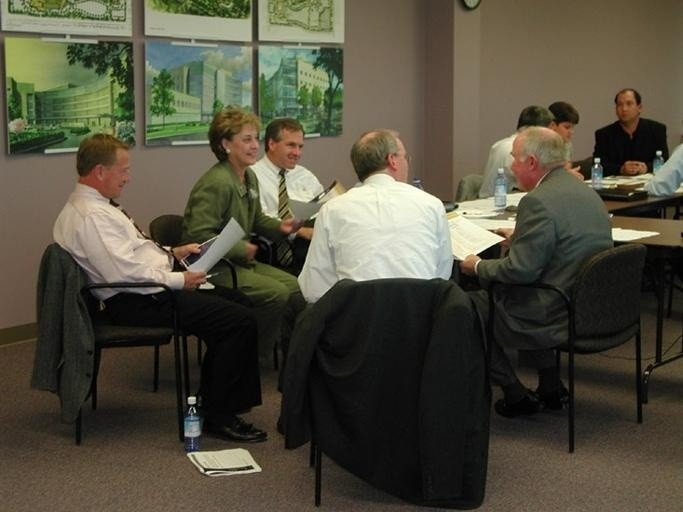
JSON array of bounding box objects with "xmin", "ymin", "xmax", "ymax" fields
[
  {"xmin": 248, "ymin": 117, "xmax": 324, "ymax": 277},
  {"xmin": 176, "ymin": 104, "xmax": 305, "ymax": 343},
  {"xmin": 48, "ymin": 133, "xmax": 267, "ymax": 443},
  {"xmin": 545, "ymin": 102, "xmax": 580, "ymax": 168},
  {"xmin": 592, "ymin": 87, "xmax": 669, "ymax": 174},
  {"xmin": 643, "ymin": 140, "xmax": 683, "ymax": 201},
  {"xmin": 297, "ymin": 128, "xmax": 455, "ymax": 305},
  {"xmin": 457, "ymin": 126, "xmax": 617, "ymax": 420},
  {"xmin": 477, "ymin": 104, "xmax": 586, "ymax": 198}
]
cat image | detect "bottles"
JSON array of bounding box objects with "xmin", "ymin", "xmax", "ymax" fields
[
  {"xmin": 652, "ymin": 150, "xmax": 663, "ymax": 175},
  {"xmin": 411, "ymin": 176, "xmax": 422, "ymax": 191},
  {"xmin": 590, "ymin": 156, "xmax": 603, "ymax": 190},
  {"xmin": 493, "ymin": 167, "xmax": 506, "ymax": 208},
  {"xmin": 182, "ymin": 396, "xmax": 201, "ymax": 453}
]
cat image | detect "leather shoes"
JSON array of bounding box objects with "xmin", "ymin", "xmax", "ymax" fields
[
  {"xmin": 206, "ymin": 408, "xmax": 267, "ymax": 443},
  {"xmin": 535, "ymin": 387, "xmax": 570, "ymax": 410},
  {"xmin": 495, "ymin": 389, "xmax": 547, "ymax": 417}
]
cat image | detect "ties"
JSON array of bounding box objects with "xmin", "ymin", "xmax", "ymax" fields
[
  {"xmin": 110, "ymin": 198, "xmax": 183, "ymax": 271},
  {"xmin": 276, "ymin": 169, "xmax": 294, "ymax": 270}
]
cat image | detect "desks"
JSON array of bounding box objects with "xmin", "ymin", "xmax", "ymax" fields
[
  {"xmin": 299, "ymin": 203, "xmax": 507, "ymax": 254},
  {"xmin": 480, "ymin": 173, "xmax": 683, "ymax": 315},
  {"xmin": 606, "ymin": 213, "xmax": 683, "ymax": 406}
]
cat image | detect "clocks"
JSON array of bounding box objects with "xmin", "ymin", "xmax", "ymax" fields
[{"xmin": 462, "ymin": 0, "xmax": 481, "ymax": 11}]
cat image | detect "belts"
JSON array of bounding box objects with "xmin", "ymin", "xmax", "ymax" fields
[{"xmin": 104, "ymin": 292, "xmax": 127, "ymax": 306}]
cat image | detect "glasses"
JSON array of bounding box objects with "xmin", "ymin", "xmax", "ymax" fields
[{"xmin": 101, "ymin": 164, "xmax": 129, "ymax": 177}]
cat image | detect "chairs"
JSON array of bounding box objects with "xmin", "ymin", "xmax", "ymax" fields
[
  {"xmin": 306, "ymin": 274, "xmax": 480, "ymax": 506},
  {"xmin": 48, "ymin": 242, "xmax": 189, "ymax": 447},
  {"xmin": 149, "ymin": 214, "xmax": 280, "ymax": 393},
  {"xmin": 486, "ymin": 242, "xmax": 646, "ymax": 453},
  {"xmin": 450, "ymin": 172, "xmax": 492, "ymax": 203}
]
